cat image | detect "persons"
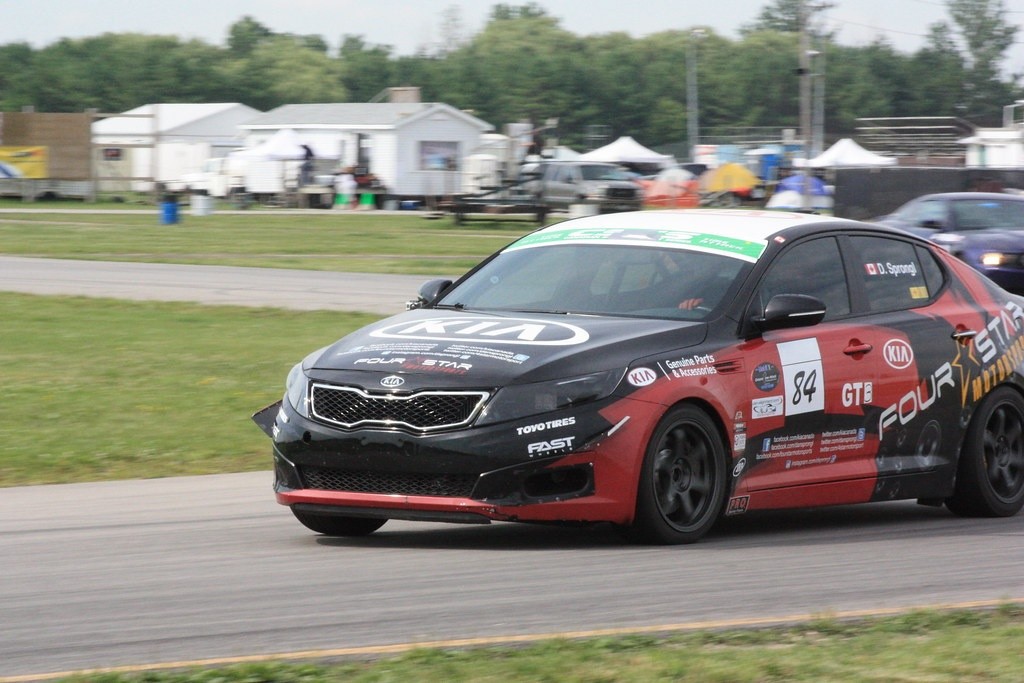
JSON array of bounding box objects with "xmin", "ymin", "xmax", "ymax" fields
[{"xmin": 630, "ymin": 254, "xmax": 736, "ymax": 310}]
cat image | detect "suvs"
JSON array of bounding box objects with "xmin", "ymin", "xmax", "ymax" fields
[{"xmin": 530, "ymin": 161, "xmax": 642, "ymax": 215}]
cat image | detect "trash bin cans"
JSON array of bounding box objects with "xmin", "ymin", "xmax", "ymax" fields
[
  {"xmin": 162, "ymin": 193, "xmax": 178, "ymax": 224},
  {"xmin": 189, "ymin": 188, "xmax": 208, "ymax": 218}
]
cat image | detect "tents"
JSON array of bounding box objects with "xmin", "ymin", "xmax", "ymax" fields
[{"xmin": 577, "ymin": 136, "xmax": 896, "ymax": 212}]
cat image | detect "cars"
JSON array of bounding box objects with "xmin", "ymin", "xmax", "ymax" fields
[
  {"xmin": 187, "ymin": 157, "xmax": 226, "ymax": 196},
  {"xmin": 1, "ymin": 163, "xmax": 47, "ymax": 204},
  {"xmin": 271, "ymin": 209, "xmax": 1023, "ymax": 546},
  {"xmin": 863, "ymin": 191, "xmax": 1024, "ymax": 292}
]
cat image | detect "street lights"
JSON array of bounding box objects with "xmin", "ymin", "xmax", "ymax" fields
[{"xmin": 685, "ymin": 30, "xmax": 707, "ymax": 162}]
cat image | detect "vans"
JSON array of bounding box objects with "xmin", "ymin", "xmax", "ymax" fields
[{"xmin": 220, "ymin": 150, "xmax": 250, "ymax": 194}]
